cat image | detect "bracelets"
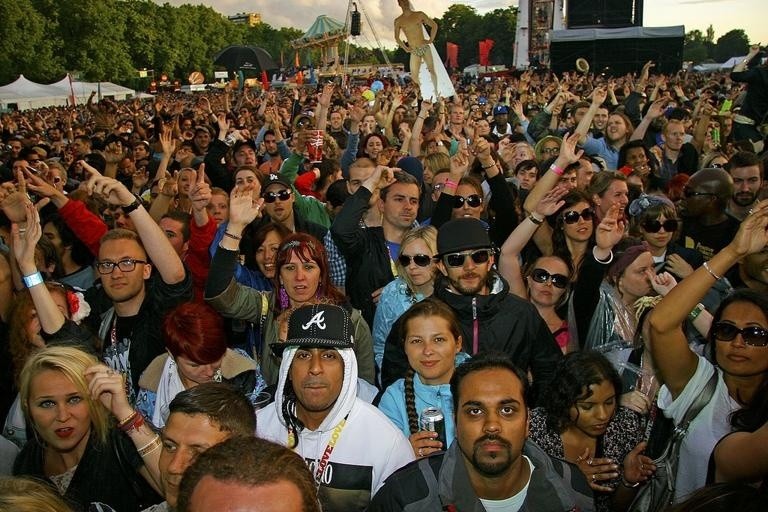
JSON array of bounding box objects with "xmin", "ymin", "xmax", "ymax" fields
[{"xmin": 742, "ymin": 57, "xmax": 750, "ymax": 65}]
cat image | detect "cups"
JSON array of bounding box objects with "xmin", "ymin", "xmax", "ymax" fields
[
  {"xmin": 635, "ymin": 369, "xmax": 658, "ymax": 403},
  {"xmin": 307, "ymin": 129, "xmax": 325, "ymax": 163}
]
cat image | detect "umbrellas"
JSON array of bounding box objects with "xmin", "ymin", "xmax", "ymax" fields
[{"xmin": 214, "ymin": 44, "xmax": 275, "ymax": 76}]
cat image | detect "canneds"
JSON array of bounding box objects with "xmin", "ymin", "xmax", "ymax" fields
[
  {"xmin": 420, "ymin": 407, "xmax": 447, "ymax": 451},
  {"xmin": 710, "ymin": 128, "xmax": 720, "ymax": 144}
]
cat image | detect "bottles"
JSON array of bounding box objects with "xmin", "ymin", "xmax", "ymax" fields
[{"xmin": 711, "ymin": 128, "xmax": 721, "ymax": 147}]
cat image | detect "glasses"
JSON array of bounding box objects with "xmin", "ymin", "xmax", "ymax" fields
[
  {"xmin": 443, "ymin": 249, "xmax": 495, "ymax": 268},
  {"xmin": 709, "ymin": 323, "xmax": 767, "ymax": 346},
  {"xmin": 682, "ymin": 185, "xmax": 720, "ymax": 199},
  {"xmin": 733, "ymin": 88, "xmax": 741, "ymax": 91},
  {"xmin": 96, "ymin": 259, "xmax": 147, "ymax": 274},
  {"xmin": 560, "ymin": 208, "xmax": 597, "ymax": 224},
  {"xmin": 262, "ymin": 190, "xmax": 293, "ymax": 203},
  {"xmin": 478, "ymin": 103, "xmax": 486, "ymax": 106},
  {"xmin": 540, "ymin": 147, "xmax": 560, "ymax": 154},
  {"xmin": 297, "ymin": 120, "xmax": 311, "ymax": 127},
  {"xmin": 470, "ymin": 98, "xmax": 478, "ymax": 101},
  {"xmin": 710, "ymin": 163, "xmax": 731, "ymax": 171},
  {"xmin": 528, "ymin": 269, "xmax": 570, "ymax": 288},
  {"xmin": 471, "ymin": 108, "xmax": 480, "ymax": 112},
  {"xmin": 641, "ymin": 218, "xmax": 678, "ymax": 233},
  {"xmin": 398, "ymin": 254, "xmax": 432, "ymax": 267},
  {"xmin": 54, "ymin": 176, "xmax": 62, "ymax": 184},
  {"xmin": 27, "ymin": 159, "xmax": 39, "ymax": 164}
]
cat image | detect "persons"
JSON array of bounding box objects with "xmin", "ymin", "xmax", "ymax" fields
[
  {"xmin": 393, "ymin": 1, "xmax": 440, "ymax": 99},
  {"xmin": 727, "ymin": 40, "xmax": 768, "ymax": 122},
  {"xmin": 0, "ymin": 61, "xmax": 767, "ymax": 511}
]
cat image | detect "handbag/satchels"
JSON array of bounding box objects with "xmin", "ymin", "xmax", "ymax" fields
[{"xmin": 611, "ymin": 433, "xmax": 678, "ymax": 512}]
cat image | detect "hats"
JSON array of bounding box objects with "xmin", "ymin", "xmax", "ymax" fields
[
  {"xmin": 136, "ymin": 140, "xmax": 149, "ymax": 147},
  {"xmin": 534, "ymin": 135, "xmax": 563, "ymax": 162},
  {"xmin": 479, "ymin": 97, "xmax": 486, "ymax": 103},
  {"xmin": 293, "ymin": 112, "xmax": 317, "ymax": 129},
  {"xmin": 261, "ymin": 173, "xmax": 292, "ymax": 194},
  {"xmin": 433, "ymin": 217, "xmax": 501, "ymax": 258},
  {"xmin": 268, "ymin": 304, "xmax": 355, "ymax": 358},
  {"xmin": 232, "ymin": 138, "xmax": 256, "ymax": 155},
  {"xmin": 576, "ymin": 57, "xmax": 589, "ymax": 73},
  {"xmin": 494, "ymin": 105, "xmax": 508, "ymax": 115}
]
